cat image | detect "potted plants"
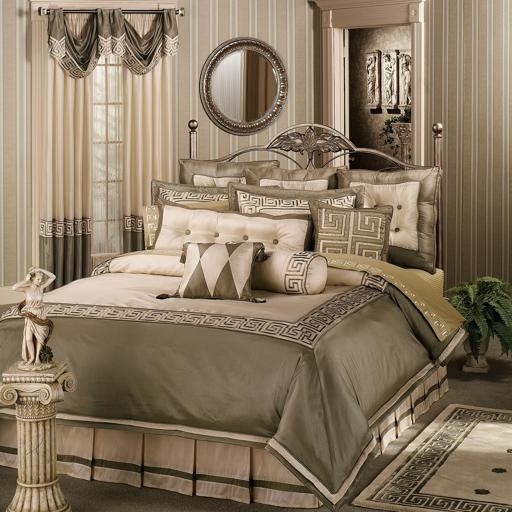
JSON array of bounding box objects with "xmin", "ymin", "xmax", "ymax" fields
[{"xmin": 444, "ymin": 275, "xmax": 512, "ymax": 373}]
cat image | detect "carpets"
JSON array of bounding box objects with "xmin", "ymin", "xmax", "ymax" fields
[{"xmin": 350, "ymin": 401, "xmax": 511, "ymax": 510}]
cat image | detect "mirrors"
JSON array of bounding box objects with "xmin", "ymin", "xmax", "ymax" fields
[{"xmin": 198, "ymin": 37, "xmax": 290, "ymax": 137}]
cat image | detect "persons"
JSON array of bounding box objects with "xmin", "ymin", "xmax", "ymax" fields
[
  {"xmin": 366, "ymin": 55, "xmax": 376, "ymax": 103},
  {"xmin": 382, "ymin": 54, "xmax": 395, "ymax": 106},
  {"xmin": 13, "ymin": 266, "xmax": 56, "ymax": 366},
  {"xmin": 399, "ymin": 54, "xmax": 411, "ymax": 106}
]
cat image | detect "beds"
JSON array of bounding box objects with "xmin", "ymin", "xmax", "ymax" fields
[{"xmin": 2, "ymin": 119, "xmax": 468, "ymax": 509}]
